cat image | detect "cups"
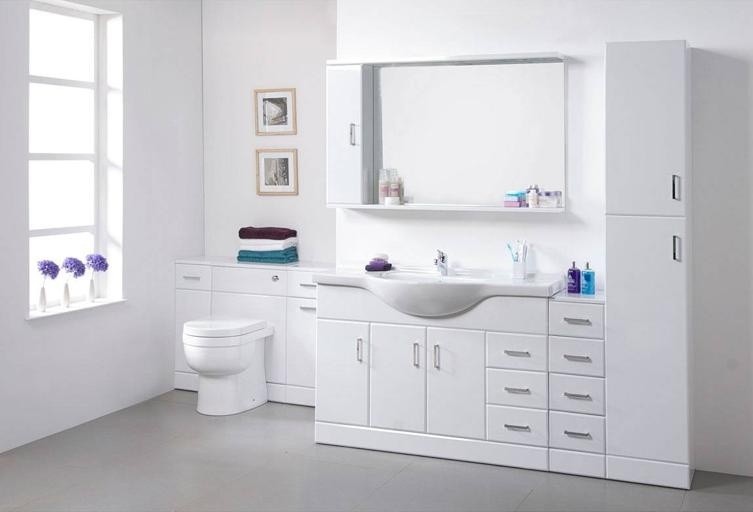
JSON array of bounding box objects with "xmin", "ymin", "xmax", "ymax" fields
[
  {"xmin": 385, "ymin": 196, "xmax": 400, "ymax": 205},
  {"xmin": 514, "ymin": 262, "xmax": 526, "ymax": 278}
]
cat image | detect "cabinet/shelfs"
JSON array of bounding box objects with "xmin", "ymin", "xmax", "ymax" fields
[
  {"xmin": 325, "ymin": 60, "xmax": 374, "ymax": 210},
  {"xmin": 486, "ymin": 332, "xmax": 549, "ymax": 449},
  {"xmin": 174, "ymin": 264, "xmax": 212, "ymax": 391},
  {"xmin": 314, "ymin": 318, "xmax": 370, "ymax": 427},
  {"xmin": 548, "ymin": 303, "xmax": 605, "ymax": 456},
  {"xmin": 369, "ymin": 323, "xmax": 486, "ymax": 440},
  {"xmin": 604, "ymin": 39, "xmax": 692, "ymax": 215},
  {"xmin": 289, "ymin": 272, "xmax": 318, "ymax": 389},
  {"xmin": 606, "ymin": 215, "xmax": 696, "ymax": 492}
]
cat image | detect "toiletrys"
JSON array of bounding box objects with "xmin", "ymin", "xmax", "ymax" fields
[
  {"xmin": 390, "ymin": 176, "xmax": 399, "ymax": 198},
  {"xmin": 526, "ymin": 184, "xmax": 561, "ymax": 207},
  {"xmin": 518, "ymin": 239, "xmax": 528, "ymax": 263},
  {"xmin": 378, "ymin": 169, "xmax": 389, "ymax": 203}
]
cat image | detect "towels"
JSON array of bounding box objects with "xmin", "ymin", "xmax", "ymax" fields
[{"xmin": 237, "ymin": 226, "xmax": 299, "ymax": 264}]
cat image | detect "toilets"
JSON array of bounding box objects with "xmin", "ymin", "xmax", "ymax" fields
[{"xmin": 182, "ymin": 319, "xmax": 276, "ymax": 416}]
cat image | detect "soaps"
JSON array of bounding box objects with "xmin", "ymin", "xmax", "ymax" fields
[{"xmin": 370, "ymin": 253, "xmax": 389, "ymax": 261}]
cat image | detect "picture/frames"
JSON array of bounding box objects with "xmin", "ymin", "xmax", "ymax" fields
[
  {"xmin": 253, "ymin": 87, "xmax": 297, "ymax": 135},
  {"xmin": 256, "ymin": 148, "xmax": 298, "ymax": 197}
]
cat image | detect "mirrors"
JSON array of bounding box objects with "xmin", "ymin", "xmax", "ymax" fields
[{"xmin": 372, "ymin": 62, "xmax": 567, "ymax": 206}]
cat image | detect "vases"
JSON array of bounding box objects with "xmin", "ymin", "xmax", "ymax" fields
[
  {"xmin": 63, "ymin": 283, "xmax": 70, "ymax": 307},
  {"xmin": 39, "ymin": 287, "xmax": 46, "ymax": 312},
  {"xmin": 89, "ymin": 279, "xmax": 95, "ymax": 303}
]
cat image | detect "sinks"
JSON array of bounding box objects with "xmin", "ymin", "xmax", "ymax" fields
[{"xmin": 372, "ymin": 269, "xmax": 490, "ymax": 317}]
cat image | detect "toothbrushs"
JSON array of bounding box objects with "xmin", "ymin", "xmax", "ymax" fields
[{"xmin": 506, "ymin": 241, "xmax": 515, "ymax": 261}]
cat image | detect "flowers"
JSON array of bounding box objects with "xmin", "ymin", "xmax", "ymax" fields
[
  {"xmin": 86, "ymin": 253, "xmax": 110, "ymax": 277},
  {"xmin": 37, "ymin": 260, "xmax": 60, "ymax": 287},
  {"xmin": 61, "ymin": 257, "xmax": 86, "ymax": 281}
]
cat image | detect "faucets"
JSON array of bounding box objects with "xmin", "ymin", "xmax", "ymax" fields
[{"xmin": 433, "ymin": 248, "xmax": 448, "ymax": 276}]
